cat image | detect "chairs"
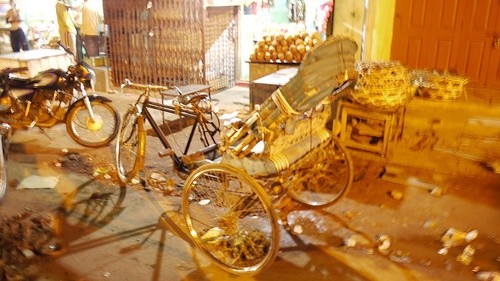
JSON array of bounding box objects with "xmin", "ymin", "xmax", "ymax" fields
[{"xmin": 188, "ymin": 35, "xmax": 362, "ymax": 178}]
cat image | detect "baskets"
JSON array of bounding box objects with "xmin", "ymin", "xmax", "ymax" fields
[
  {"xmin": 351, "ymin": 57, "xmax": 415, "ymax": 111},
  {"xmin": 410, "ymin": 65, "xmax": 467, "ymax": 101}
]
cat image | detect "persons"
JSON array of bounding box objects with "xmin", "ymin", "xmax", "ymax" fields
[
  {"xmin": 56, "ymin": 0, "xmax": 79, "ymax": 59},
  {"xmin": 6, "ymin": 0, "xmax": 29, "ymax": 52},
  {"xmin": 74, "ymin": 0, "xmax": 105, "ymax": 57}
]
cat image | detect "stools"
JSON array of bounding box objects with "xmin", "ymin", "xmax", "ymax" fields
[{"xmin": 159, "ymin": 84, "xmax": 213, "ymax": 130}]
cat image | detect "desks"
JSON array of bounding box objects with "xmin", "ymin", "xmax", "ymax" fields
[
  {"xmin": 248, "ymin": 65, "xmax": 300, "ymax": 112},
  {"xmin": 1, "ymin": 49, "xmax": 71, "ymax": 78},
  {"xmin": 244, "ymin": 59, "xmax": 301, "ymax": 82},
  {"xmin": 339, "ymin": 102, "xmax": 406, "ymax": 158}
]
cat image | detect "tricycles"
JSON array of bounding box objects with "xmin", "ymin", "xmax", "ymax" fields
[{"xmin": 114, "ymin": 35, "xmax": 361, "ymax": 275}]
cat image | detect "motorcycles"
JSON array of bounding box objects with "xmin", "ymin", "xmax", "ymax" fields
[{"xmin": 0, "ymin": 32, "xmax": 120, "ymax": 149}]
